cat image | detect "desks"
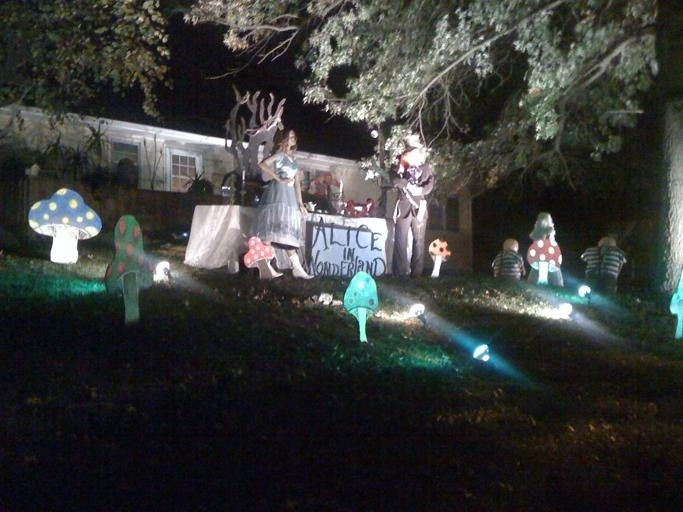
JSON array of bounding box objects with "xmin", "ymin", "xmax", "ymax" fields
[{"xmin": 184, "ymin": 204, "xmax": 388, "ymax": 277}]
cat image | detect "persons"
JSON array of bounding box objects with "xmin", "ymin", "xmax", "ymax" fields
[
  {"xmin": 485, "ymin": 236, "xmax": 528, "ymax": 280},
  {"xmin": 259, "ymin": 129, "xmax": 319, "ymax": 280},
  {"xmin": 581, "ymin": 236, "xmax": 627, "ymax": 294},
  {"xmin": 384, "ymin": 138, "xmax": 436, "ymax": 276}
]
